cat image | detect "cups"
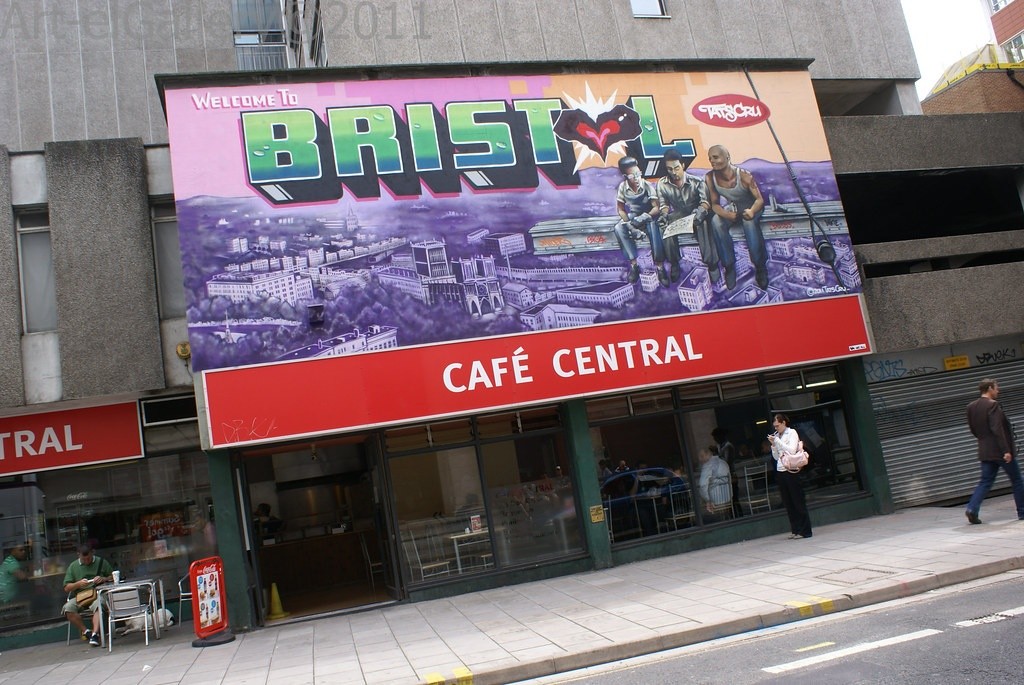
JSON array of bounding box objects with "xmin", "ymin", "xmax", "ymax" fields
[{"xmin": 112, "ymin": 571, "xmax": 120, "ymax": 584}]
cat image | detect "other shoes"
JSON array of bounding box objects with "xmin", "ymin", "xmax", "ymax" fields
[
  {"xmin": 788, "ymin": 532, "xmax": 796, "ymax": 539},
  {"xmin": 90, "ymin": 633, "xmax": 99, "ymax": 646},
  {"xmin": 84, "ymin": 632, "xmax": 91, "ymax": 639},
  {"xmin": 795, "ymin": 534, "xmax": 803, "ymax": 539},
  {"xmin": 966, "ymin": 511, "xmax": 982, "ymax": 524}
]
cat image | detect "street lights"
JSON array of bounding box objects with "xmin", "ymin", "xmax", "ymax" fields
[{"xmin": 39, "ymin": 509, "xmax": 48, "ymax": 548}]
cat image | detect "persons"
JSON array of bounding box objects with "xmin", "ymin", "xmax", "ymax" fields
[
  {"xmin": 61, "ymin": 545, "xmax": 113, "ymax": 646},
  {"xmin": 194, "ymin": 512, "xmax": 217, "ymax": 558},
  {"xmin": 766, "ymin": 414, "xmax": 813, "ymax": 538},
  {"xmin": 659, "ymin": 470, "xmax": 691, "ymax": 517},
  {"xmin": 965, "ymin": 378, "xmax": 1024, "ymax": 524},
  {"xmin": 252, "ymin": 503, "xmax": 282, "ymax": 542},
  {"xmin": 709, "ymin": 427, "xmax": 743, "ymax": 518},
  {"xmin": 615, "ymin": 460, "xmax": 629, "ymax": 473},
  {"xmin": 599, "ymin": 459, "xmax": 612, "ymax": 477},
  {"xmin": 0, "ymin": 542, "xmax": 43, "ymax": 621},
  {"xmin": 697, "ymin": 446, "xmax": 733, "ymax": 513}
]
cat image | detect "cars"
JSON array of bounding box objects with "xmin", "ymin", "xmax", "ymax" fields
[
  {"xmin": 52, "ymin": 512, "xmax": 94, "ymax": 549},
  {"xmin": 532, "ymin": 466, "xmax": 690, "ymax": 551},
  {"xmin": 454, "ymin": 504, "xmax": 487, "ymax": 521}
]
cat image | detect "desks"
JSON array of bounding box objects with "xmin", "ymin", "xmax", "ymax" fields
[
  {"xmin": 137, "ymin": 548, "xmax": 193, "ymax": 596},
  {"xmin": 637, "ymin": 492, "xmax": 675, "ymax": 533},
  {"xmin": 27, "ymin": 569, "xmax": 68, "ymax": 619},
  {"xmin": 441, "ymin": 526, "xmax": 509, "ymax": 573},
  {"xmin": 96, "ymin": 573, "xmax": 167, "ymax": 645}
]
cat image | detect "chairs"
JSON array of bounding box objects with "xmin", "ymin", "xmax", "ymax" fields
[
  {"xmin": 358, "ymin": 463, "xmax": 773, "ymax": 594},
  {"xmin": 66, "ymin": 572, "xmax": 192, "ymax": 653}
]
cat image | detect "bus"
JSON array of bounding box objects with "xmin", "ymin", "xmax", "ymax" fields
[{"xmin": 84, "ymin": 499, "xmax": 198, "ymax": 548}]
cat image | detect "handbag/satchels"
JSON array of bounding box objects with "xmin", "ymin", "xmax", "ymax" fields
[
  {"xmin": 780, "ymin": 441, "xmax": 809, "ymax": 474},
  {"xmin": 76, "ymin": 588, "xmax": 97, "ymax": 607}
]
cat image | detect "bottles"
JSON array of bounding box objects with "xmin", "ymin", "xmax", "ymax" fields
[
  {"xmin": 217, "ymin": 602, "xmax": 219, "ymax": 615},
  {"xmin": 206, "ymin": 604, "xmax": 208, "ymax": 619},
  {"xmin": 204, "ymin": 578, "xmax": 207, "ymax": 593},
  {"xmin": 215, "ymin": 575, "xmax": 218, "ymax": 590}
]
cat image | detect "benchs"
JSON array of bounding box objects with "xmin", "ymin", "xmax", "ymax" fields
[{"xmin": 0, "ymin": 599, "xmax": 50, "ymax": 627}]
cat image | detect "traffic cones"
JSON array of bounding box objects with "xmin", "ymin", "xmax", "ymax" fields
[{"xmin": 267, "ymin": 583, "xmax": 290, "ymax": 619}]
